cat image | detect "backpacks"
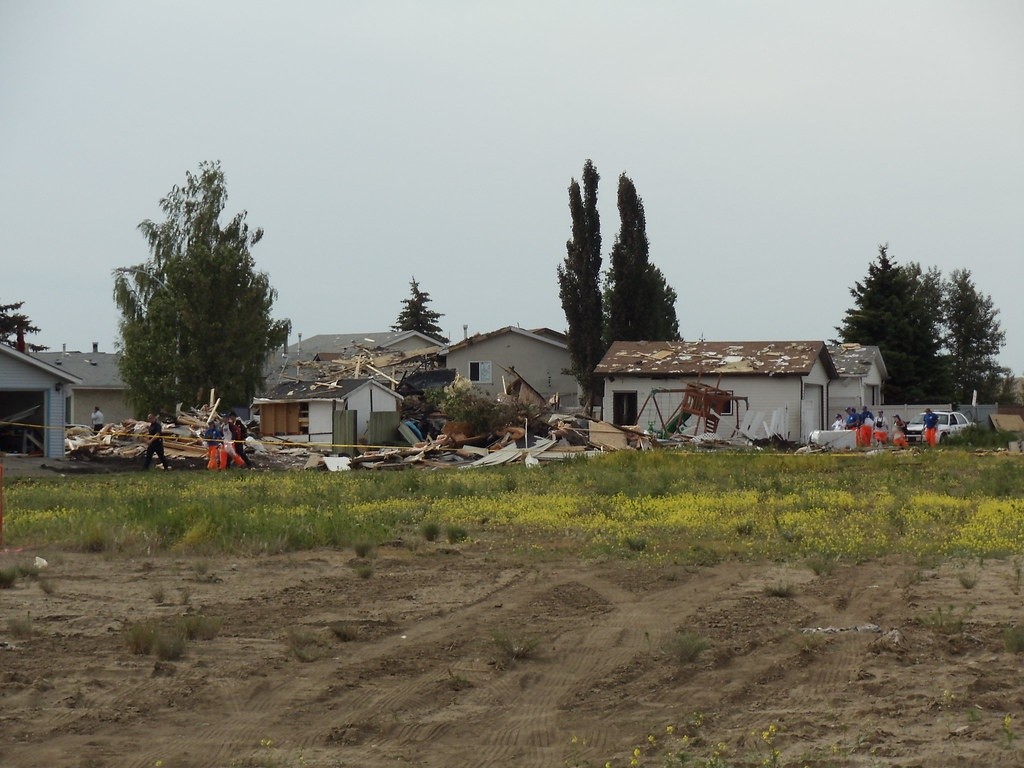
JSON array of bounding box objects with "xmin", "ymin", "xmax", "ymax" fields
[
  {"xmin": 901, "ymin": 421, "xmax": 908, "ymax": 432},
  {"xmin": 232, "ymin": 419, "xmax": 249, "ymax": 438}
]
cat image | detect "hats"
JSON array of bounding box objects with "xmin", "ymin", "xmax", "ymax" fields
[
  {"xmin": 892, "ymin": 414, "xmax": 899, "ymax": 417},
  {"xmin": 878, "ymin": 410, "xmax": 883, "ymax": 413},
  {"xmin": 835, "ymin": 414, "xmax": 841, "ymax": 418},
  {"xmin": 923, "ymin": 408, "xmax": 932, "ymax": 412},
  {"xmin": 844, "ymin": 407, "xmax": 850, "ymax": 411}
]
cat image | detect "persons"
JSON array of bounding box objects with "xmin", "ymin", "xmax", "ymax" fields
[
  {"xmin": 91, "ymin": 406, "xmax": 104, "ymax": 435},
  {"xmin": 831, "ymin": 406, "xmax": 874, "ymax": 447},
  {"xmin": 142, "ymin": 414, "xmax": 167, "ymax": 472},
  {"xmin": 892, "ymin": 414, "xmax": 908, "ymax": 447},
  {"xmin": 874, "ymin": 410, "xmax": 889, "ymax": 444},
  {"xmin": 923, "ymin": 408, "xmax": 938, "ymax": 447},
  {"xmin": 204, "ymin": 411, "xmax": 251, "ymax": 470}
]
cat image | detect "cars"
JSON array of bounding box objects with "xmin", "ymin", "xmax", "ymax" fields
[{"xmin": 904, "ymin": 411, "xmax": 976, "ymax": 445}]
